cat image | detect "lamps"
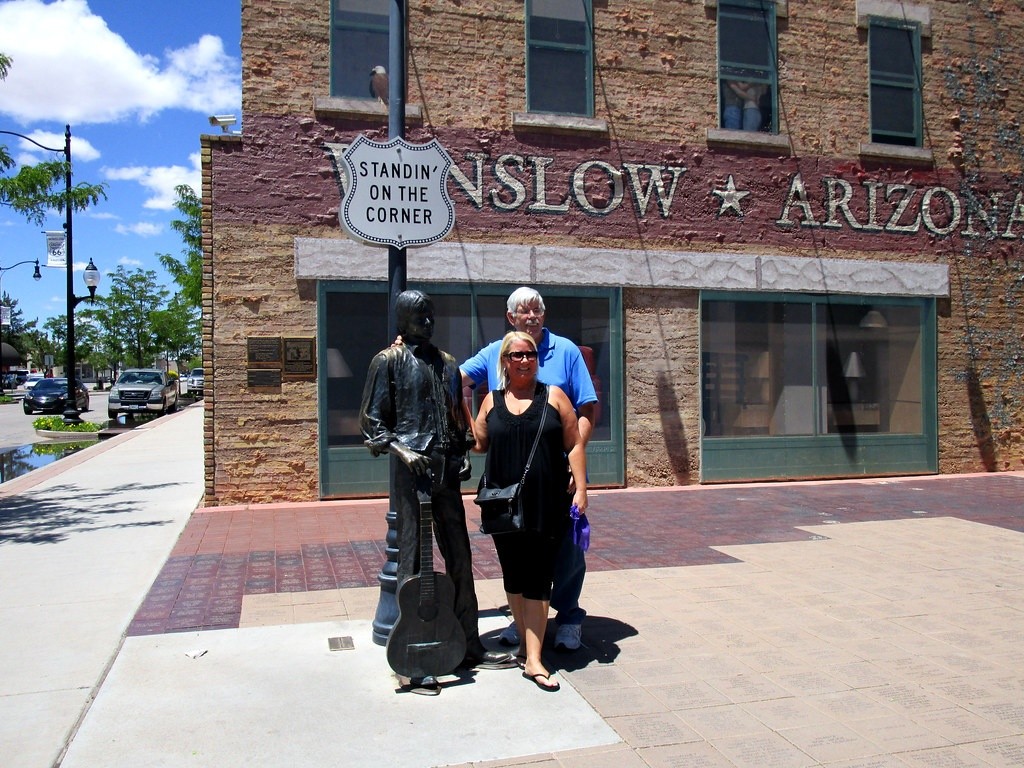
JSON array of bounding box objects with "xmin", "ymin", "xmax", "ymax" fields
[
  {"xmin": 828, "ymin": 403, "xmax": 881, "ymax": 426},
  {"xmin": 748, "ymin": 351, "xmax": 769, "ymax": 403},
  {"xmin": 842, "ymin": 352, "xmax": 868, "ymax": 403},
  {"xmin": 859, "ymin": 310, "xmax": 888, "ymax": 328}
]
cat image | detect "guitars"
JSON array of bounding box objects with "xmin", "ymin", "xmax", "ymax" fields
[{"xmin": 387, "ymin": 461, "xmax": 465, "ymax": 681}]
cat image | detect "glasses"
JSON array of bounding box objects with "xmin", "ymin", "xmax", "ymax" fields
[
  {"xmin": 504, "ymin": 350, "xmax": 538, "ymax": 362},
  {"xmin": 518, "ymin": 308, "xmax": 544, "ymax": 317}
]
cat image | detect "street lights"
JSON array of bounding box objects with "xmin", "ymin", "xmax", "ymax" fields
[
  {"xmin": 65, "ymin": 256, "xmax": 102, "ymax": 425},
  {"xmin": 0, "ymin": 259, "xmax": 41, "ymax": 283}
]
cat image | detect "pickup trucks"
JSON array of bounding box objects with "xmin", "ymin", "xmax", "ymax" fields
[
  {"xmin": 187, "ymin": 367, "xmax": 204, "ymax": 395},
  {"xmin": 107, "ymin": 367, "xmax": 177, "ymax": 419}
]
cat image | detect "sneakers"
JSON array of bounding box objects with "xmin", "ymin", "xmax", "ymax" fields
[
  {"xmin": 498, "ymin": 621, "xmax": 520, "ymax": 648},
  {"xmin": 554, "ymin": 624, "xmax": 590, "ymax": 655}
]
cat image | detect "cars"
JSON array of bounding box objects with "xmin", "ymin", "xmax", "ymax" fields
[
  {"xmin": 22, "ymin": 378, "xmax": 90, "ymax": 415},
  {"xmin": 23, "ymin": 375, "xmax": 44, "ymax": 391},
  {"xmin": 2, "ymin": 374, "xmax": 18, "ymax": 388}
]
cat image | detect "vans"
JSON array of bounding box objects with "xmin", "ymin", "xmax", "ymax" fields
[{"xmin": 9, "ymin": 370, "xmax": 29, "ymax": 383}]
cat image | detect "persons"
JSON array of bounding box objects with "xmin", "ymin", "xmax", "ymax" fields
[
  {"xmin": 44, "ymin": 366, "xmax": 54, "ymax": 379},
  {"xmin": 391, "ymin": 287, "xmax": 599, "ymax": 650},
  {"xmin": 463, "ymin": 331, "xmax": 588, "ymax": 693},
  {"xmin": 358, "ymin": 290, "xmax": 518, "ymax": 696}
]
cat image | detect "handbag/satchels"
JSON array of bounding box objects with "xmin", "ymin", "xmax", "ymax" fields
[{"xmin": 474, "ymin": 482, "xmax": 525, "ymax": 534}]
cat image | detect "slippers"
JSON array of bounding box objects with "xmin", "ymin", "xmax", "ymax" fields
[
  {"xmin": 516, "ymin": 655, "xmax": 527, "ymax": 670},
  {"xmin": 522, "ymin": 672, "xmax": 560, "ymax": 692}
]
